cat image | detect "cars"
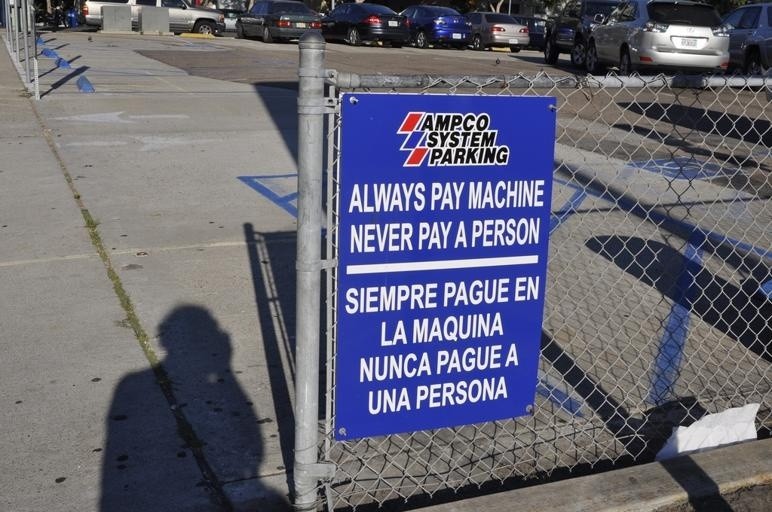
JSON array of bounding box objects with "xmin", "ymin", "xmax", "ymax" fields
[
  {"xmin": 463, "ymin": 11, "xmax": 530, "ymax": 53},
  {"xmin": 719, "ymin": 3, "xmax": 772, "ymax": 91},
  {"xmin": 582, "ymin": 1, "xmax": 730, "ymax": 75},
  {"xmin": 543, "ymin": 0, "xmax": 623, "ymax": 65},
  {"xmin": 511, "ymin": 14, "xmax": 548, "ymax": 52},
  {"xmin": 397, "ymin": 5, "xmax": 473, "ymax": 50},
  {"xmin": 83, "ymin": 0, "xmax": 226, "ymax": 35},
  {"xmin": 235, "ymin": 0, "xmax": 323, "ymax": 43},
  {"xmin": 322, "ymin": 3, "xmax": 410, "ymax": 49},
  {"xmin": 216, "ymin": 8, "xmax": 241, "ymax": 30}
]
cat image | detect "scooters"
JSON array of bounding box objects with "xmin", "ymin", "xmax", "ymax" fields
[{"xmin": 35, "ymin": 0, "xmax": 83, "ymax": 33}]
